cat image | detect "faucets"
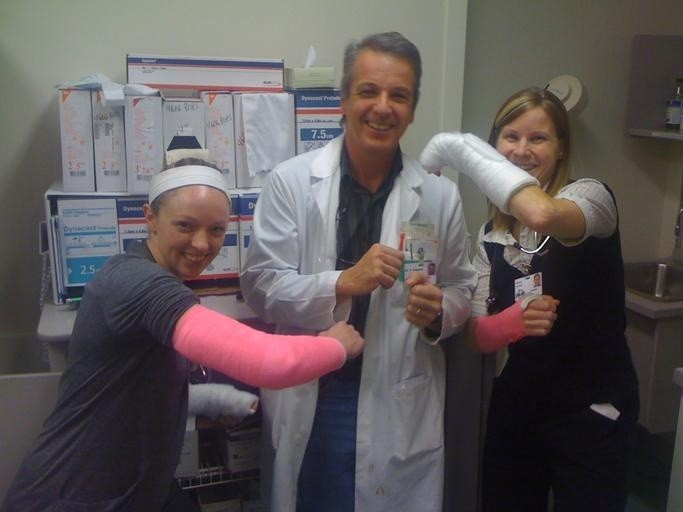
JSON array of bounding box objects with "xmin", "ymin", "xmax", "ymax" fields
[{"xmin": 652, "ymin": 261, "xmax": 670, "ymax": 299}]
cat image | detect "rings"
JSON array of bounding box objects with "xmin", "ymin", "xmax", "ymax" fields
[{"xmin": 415, "ymin": 309, "xmax": 422, "ymax": 315}]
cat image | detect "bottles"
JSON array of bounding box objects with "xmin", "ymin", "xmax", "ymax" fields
[{"xmin": 663, "ymin": 77, "xmax": 682, "ymax": 130}]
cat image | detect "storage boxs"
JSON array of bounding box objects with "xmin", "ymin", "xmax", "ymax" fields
[
  {"xmin": 41, "ymin": 52, "xmax": 351, "ymax": 301},
  {"xmin": 216, "ymin": 416, "xmax": 262, "ymax": 473},
  {"xmin": 172, "ymin": 414, "xmax": 199, "ymax": 477}
]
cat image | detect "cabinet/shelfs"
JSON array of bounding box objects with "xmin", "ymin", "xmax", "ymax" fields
[{"xmin": 36, "ymin": 288, "xmax": 499, "ymax": 511}]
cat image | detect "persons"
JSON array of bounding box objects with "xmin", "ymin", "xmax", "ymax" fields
[
  {"xmin": 239, "ymin": 31, "xmax": 479, "ymax": 512},
  {"xmin": 533, "ymin": 273, "xmax": 541, "ymax": 288},
  {"xmin": 1, "ymin": 157, "xmax": 365, "ymax": 511},
  {"xmin": 427, "ymin": 263, "xmax": 436, "ymax": 275},
  {"xmin": 419, "ymin": 88, "xmax": 639, "ymax": 511}
]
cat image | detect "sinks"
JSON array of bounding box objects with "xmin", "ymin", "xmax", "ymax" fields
[{"xmin": 622, "ymin": 261, "xmax": 683, "ymax": 304}]
